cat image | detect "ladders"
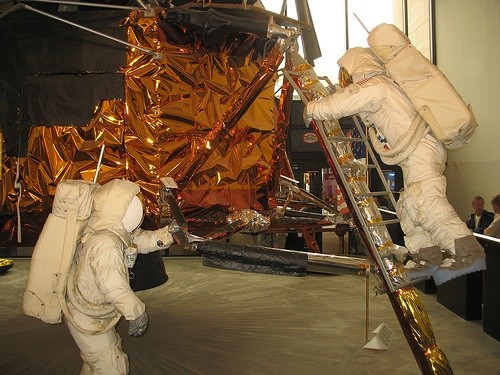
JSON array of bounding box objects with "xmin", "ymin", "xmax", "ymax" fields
[{"xmin": 282, "ymin": 68, "xmax": 431, "ymax": 293}]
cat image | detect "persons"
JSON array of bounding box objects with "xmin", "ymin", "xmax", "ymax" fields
[
  {"xmin": 63, "ymin": 179, "xmax": 178, "ymax": 375},
  {"xmin": 302, "ymin": 47, "xmax": 487, "ymax": 290},
  {"xmin": 461, "ymin": 194, "xmax": 499, "ymax": 321}
]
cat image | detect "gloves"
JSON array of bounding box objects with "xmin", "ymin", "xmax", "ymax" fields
[
  {"xmin": 129, "ymin": 312, "xmax": 149, "ymax": 336},
  {"xmin": 303, "ymin": 100, "xmax": 314, "ymax": 128},
  {"xmin": 169, "ymin": 220, "xmax": 180, "ymax": 233}
]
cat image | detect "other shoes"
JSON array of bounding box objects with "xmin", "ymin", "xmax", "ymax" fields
[
  {"xmin": 433, "ymin": 235, "xmax": 488, "ymax": 286},
  {"xmin": 403, "ymin": 247, "xmax": 442, "ymax": 278}
]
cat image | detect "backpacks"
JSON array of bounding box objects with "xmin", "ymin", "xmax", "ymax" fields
[
  {"xmin": 369, "ymin": 22, "xmax": 478, "ymax": 167},
  {"xmin": 24, "ymin": 179, "xmax": 130, "ymax": 335}
]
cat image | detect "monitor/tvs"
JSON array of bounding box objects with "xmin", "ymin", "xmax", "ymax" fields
[{"xmin": 370, "ymin": 171, "xmax": 395, "ymax": 191}]
